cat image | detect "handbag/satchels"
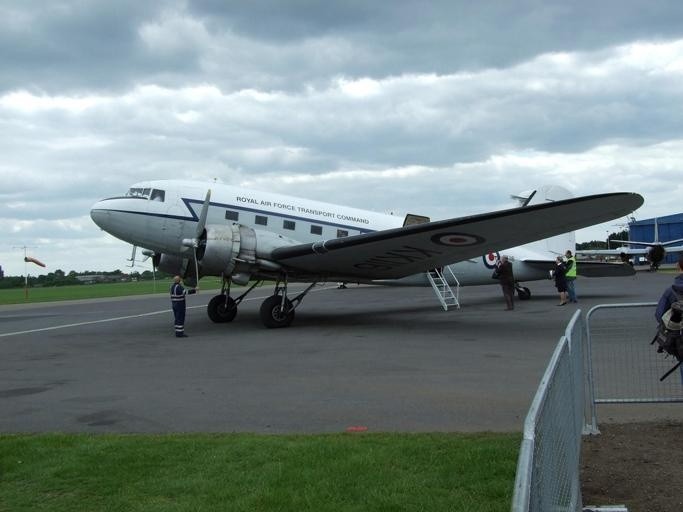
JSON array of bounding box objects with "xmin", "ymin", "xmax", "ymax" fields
[{"xmin": 654, "ymin": 300, "xmax": 682, "ymax": 362}]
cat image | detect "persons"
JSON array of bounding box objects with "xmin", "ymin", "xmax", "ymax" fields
[
  {"xmin": 491, "ymin": 254, "xmax": 515, "ymax": 312},
  {"xmin": 548, "ymin": 256, "xmax": 568, "ymax": 307},
  {"xmin": 654, "ymin": 252, "xmax": 682, "ymax": 385},
  {"xmin": 562, "ymin": 249, "xmax": 578, "ymax": 304},
  {"xmin": 168, "ymin": 275, "xmax": 198, "ymax": 338}
]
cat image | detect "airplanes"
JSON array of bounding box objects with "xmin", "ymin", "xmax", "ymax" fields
[
  {"xmin": 89, "ymin": 178, "xmax": 643, "ymax": 329},
  {"xmin": 574, "ymin": 218, "xmax": 683, "ymax": 273}
]
